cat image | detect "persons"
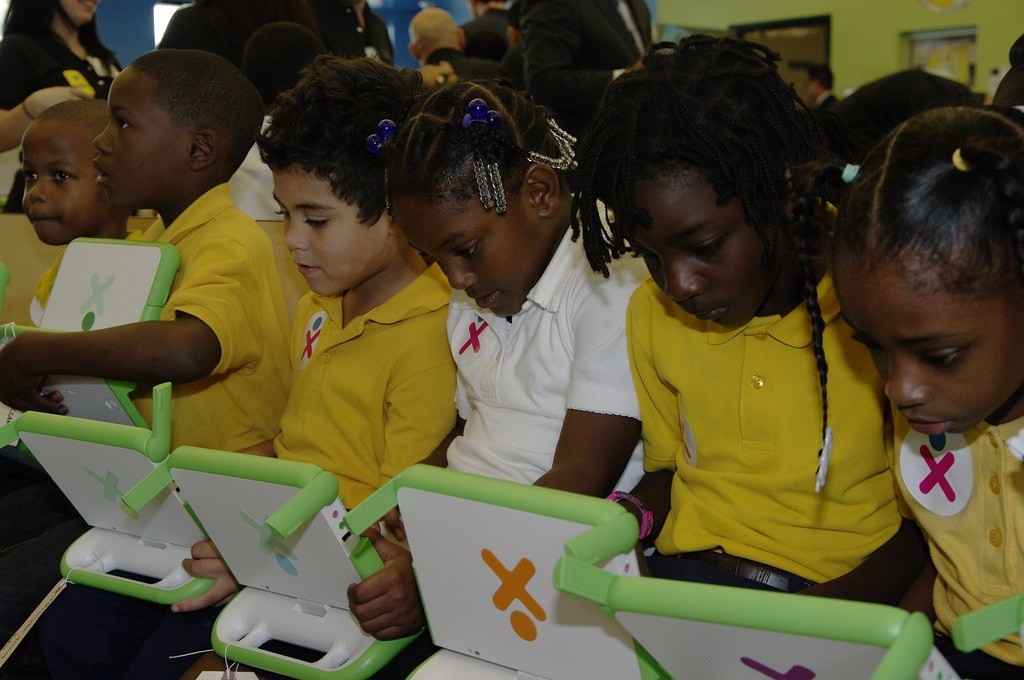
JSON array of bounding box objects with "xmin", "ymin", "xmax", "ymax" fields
[{"xmin": 0, "ymin": 0, "xmax": 1024, "ymax": 680}]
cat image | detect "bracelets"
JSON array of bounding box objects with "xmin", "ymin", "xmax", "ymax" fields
[
  {"xmin": 21, "ymin": 100, "xmax": 35, "ymax": 120},
  {"xmin": 606, "ymin": 490, "xmax": 653, "ymax": 540},
  {"xmin": 432, "ymin": 70, "xmax": 450, "ymax": 89}
]
